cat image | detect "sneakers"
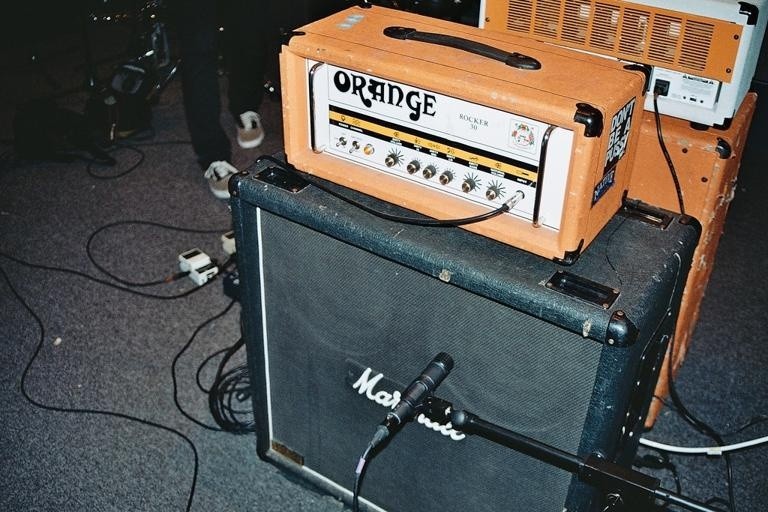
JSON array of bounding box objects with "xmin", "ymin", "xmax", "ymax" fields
[
  {"xmin": 235, "ymin": 110, "xmax": 265, "ymax": 150},
  {"xmin": 204, "ymin": 160, "xmax": 240, "ymax": 199}
]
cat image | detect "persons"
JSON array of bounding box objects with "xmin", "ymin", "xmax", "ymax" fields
[{"xmin": 161, "ymin": 0, "xmax": 266, "ymax": 200}]
